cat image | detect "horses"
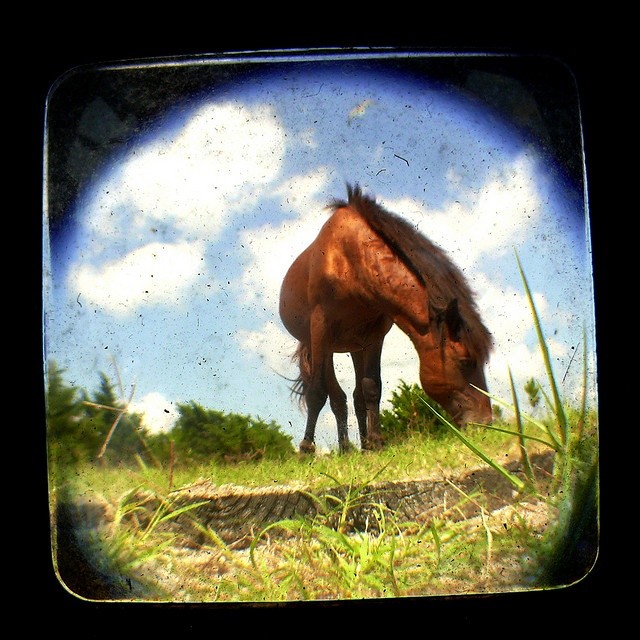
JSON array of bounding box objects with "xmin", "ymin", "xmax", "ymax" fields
[{"xmin": 278, "ymin": 189, "xmax": 496, "ymax": 467}]
